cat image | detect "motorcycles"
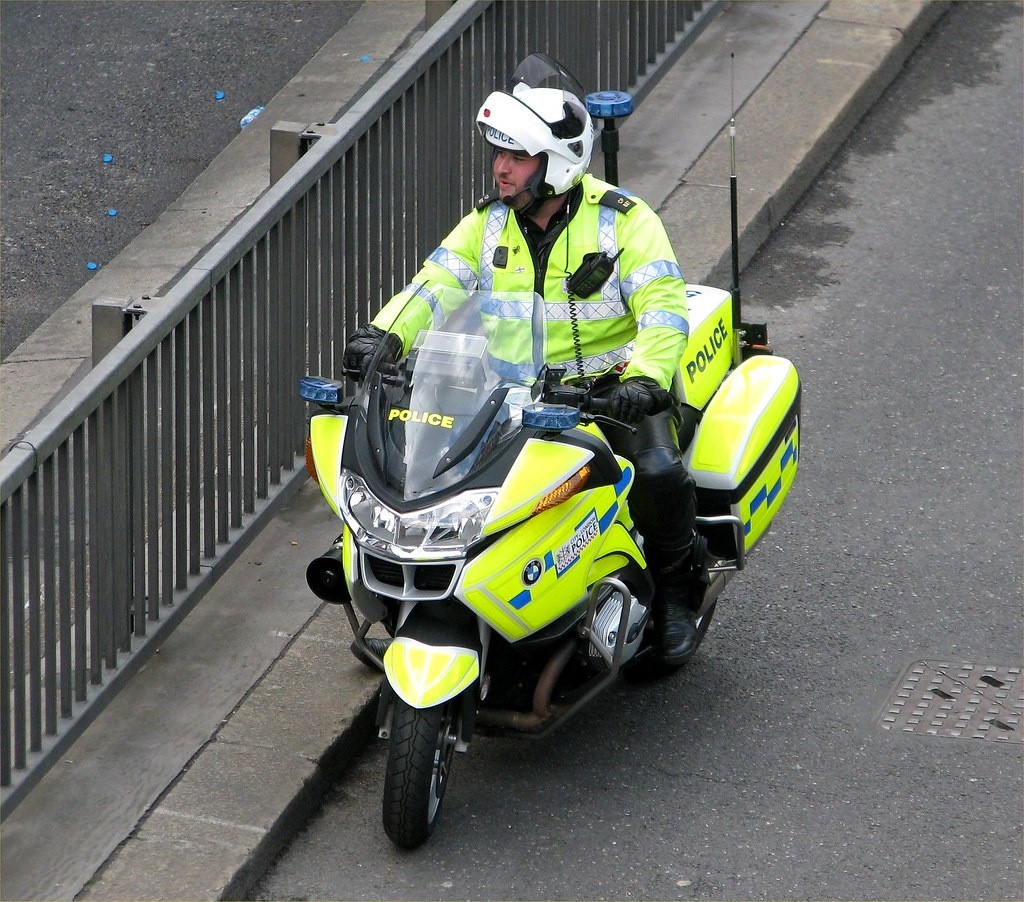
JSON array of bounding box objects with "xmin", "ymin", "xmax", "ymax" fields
[{"xmin": 299, "ymin": 52, "xmax": 803, "ymax": 850}]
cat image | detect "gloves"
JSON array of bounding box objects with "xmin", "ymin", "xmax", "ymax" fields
[
  {"xmin": 609, "ymin": 381, "xmax": 660, "ymax": 427},
  {"xmin": 343, "ymin": 322, "xmax": 389, "ymax": 381}
]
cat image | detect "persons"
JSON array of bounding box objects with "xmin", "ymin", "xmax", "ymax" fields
[{"xmin": 343, "ymin": 84, "xmax": 712, "ymax": 669}]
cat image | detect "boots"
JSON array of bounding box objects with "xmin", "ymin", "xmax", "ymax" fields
[{"xmin": 642, "ymin": 539, "xmax": 698, "ymax": 665}]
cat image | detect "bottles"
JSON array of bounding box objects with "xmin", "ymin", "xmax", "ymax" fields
[{"xmin": 241, "ymin": 105, "xmax": 264, "ymax": 127}]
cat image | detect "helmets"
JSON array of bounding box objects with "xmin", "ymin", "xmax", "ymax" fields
[{"xmin": 475, "ymin": 88, "xmax": 596, "ymax": 200}]
